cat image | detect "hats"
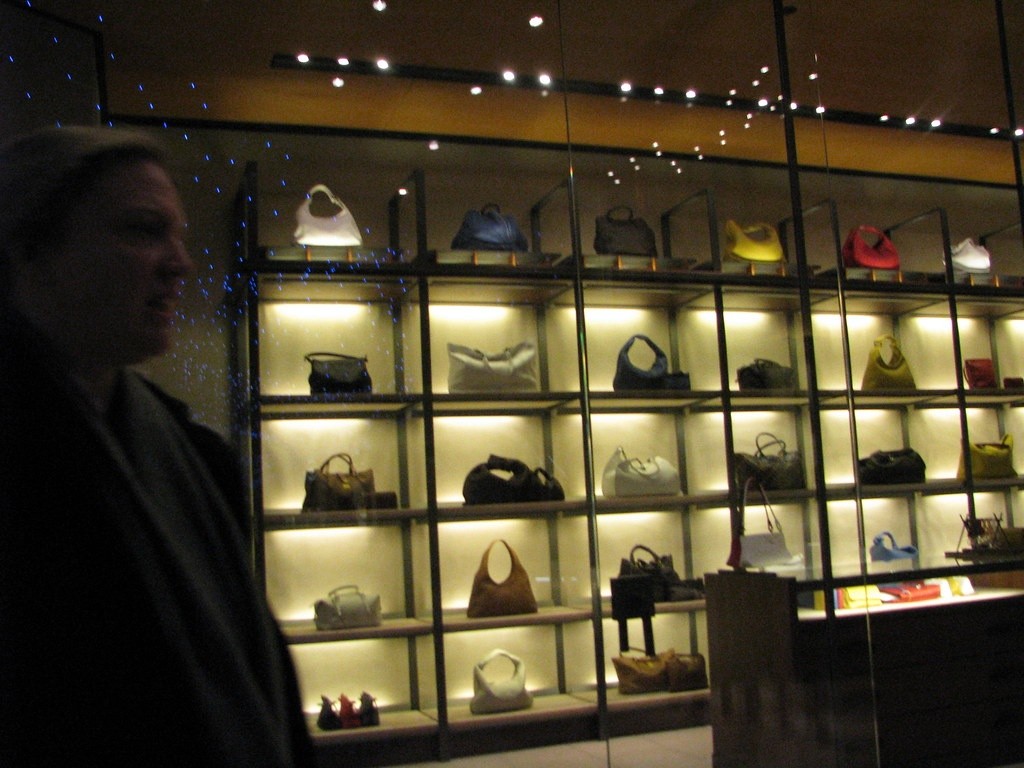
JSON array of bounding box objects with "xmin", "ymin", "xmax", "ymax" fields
[{"xmin": 0, "ymin": 127, "xmax": 174, "ymax": 234}]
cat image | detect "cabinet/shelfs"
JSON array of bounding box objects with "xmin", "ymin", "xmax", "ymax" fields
[{"xmin": 232, "ymin": 162, "xmax": 1024, "ymax": 768}]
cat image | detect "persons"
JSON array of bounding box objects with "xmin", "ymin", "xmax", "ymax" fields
[{"xmin": 0, "ymin": 125, "xmax": 320, "ymax": 768}]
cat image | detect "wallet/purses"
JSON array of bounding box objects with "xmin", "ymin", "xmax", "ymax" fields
[
  {"xmin": 811, "ymin": 575, "xmax": 975, "ymax": 611},
  {"xmin": 366, "ymin": 491, "xmax": 398, "ymax": 521}
]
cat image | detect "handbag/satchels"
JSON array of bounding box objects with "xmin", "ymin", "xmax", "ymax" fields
[
  {"xmin": 871, "ymin": 531, "xmax": 919, "ymax": 588},
  {"xmin": 724, "ymin": 218, "xmax": 786, "ymax": 263},
  {"xmin": 941, "ymin": 239, "xmax": 991, "ymax": 273},
  {"xmin": 301, "ymin": 453, "xmax": 376, "ymax": 512},
  {"xmin": 856, "ymin": 447, "xmax": 926, "ymax": 485},
  {"xmin": 736, "ymin": 431, "xmax": 810, "ymax": 492},
  {"xmin": 959, "ymin": 435, "xmax": 1019, "ymax": 481},
  {"xmin": 726, "ymin": 477, "xmax": 791, "ymax": 568},
  {"xmin": 468, "ymin": 648, "xmax": 532, "ymax": 714},
  {"xmin": 601, "ymin": 447, "xmax": 681, "ymax": 497},
  {"xmin": 964, "ymin": 358, "xmax": 999, "ymax": 389},
  {"xmin": 467, "ymin": 537, "xmax": 539, "ymax": 617},
  {"xmin": 993, "ymin": 526, "xmax": 1024, "ymax": 553},
  {"xmin": 594, "ymin": 204, "xmax": 658, "ymax": 258},
  {"xmin": 737, "ymin": 359, "xmax": 801, "ymax": 396},
  {"xmin": 617, "ymin": 544, "xmax": 677, "ymax": 601},
  {"xmin": 462, "ymin": 454, "xmax": 564, "ymax": 504},
  {"xmin": 612, "ymin": 334, "xmax": 692, "ymax": 390},
  {"xmin": 861, "ymin": 334, "xmax": 917, "ymax": 393},
  {"xmin": 842, "ymin": 226, "xmax": 900, "ymax": 270},
  {"xmin": 312, "ymin": 584, "xmax": 382, "ymax": 630},
  {"xmin": 305, "ymin": 351, "xmax": 372, "ymax": 394},
  {"xmin": 317, "ymin": 692, "xmax": 379, "ymax": 732},
  {"xmin": 449, "ymin": 203, "xmax": 528, "ymax": 251},
  {"xmin": 448, "ymin": 340, "xmax": 540, "ymax": 393},
  {"xmin": 293, "ymin": 180, "xmax": 363, "ymax": 249},
  {"xmin": 612, "ymin": 644, "xmax": 709, "ymax": 692}
]
